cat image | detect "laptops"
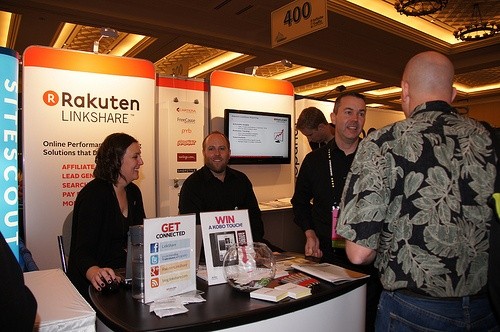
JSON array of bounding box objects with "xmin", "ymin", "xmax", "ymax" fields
[{"xmin": 114, "ymin": 225, "xmax": 202, "ymax": 284}]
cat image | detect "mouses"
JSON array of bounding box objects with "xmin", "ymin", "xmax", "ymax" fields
[{"xmin": 102, "ymin": 280, "xmax": 120, "ymax": 296}]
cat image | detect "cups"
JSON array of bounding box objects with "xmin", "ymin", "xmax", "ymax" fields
[{"xmin": 130, "ymin": 225, "xmax": 145, "ymax": 299}]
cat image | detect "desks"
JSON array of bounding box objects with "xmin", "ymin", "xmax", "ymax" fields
[{"xmin": 23, "ymin": 269, "xmax": 97, "ymax": 332}]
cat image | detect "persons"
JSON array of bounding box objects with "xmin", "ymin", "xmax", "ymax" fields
[
  {"xmin": 66, "ymin": 133, "xmax": 147, "ymax": 292},
  {"xmin": 0, "ymin": 232, "xmax": 41, "ymax": 332},
  {"xmin": 295, "ymin": 108, "xmax": 336, "ymax": 152},
  {"xmin": 290, "ymin": 92, "xmax": 367, "ymax": 259},
  {"xmin": 178, "ymin": 130, "xmax": 286, "ymax": 270},
  {"xmin": 335, "ymin": 51, "xmax": 500, "ymax": 332}
]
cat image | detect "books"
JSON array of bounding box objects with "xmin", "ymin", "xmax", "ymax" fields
[{"xmin": 289, "ymin": 262, "xmax": 371, "ymax": 285}]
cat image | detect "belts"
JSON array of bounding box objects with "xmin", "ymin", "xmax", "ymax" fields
[{"xmin": 384, "ymin": 288, "xmax": 488, "ymax": 301}]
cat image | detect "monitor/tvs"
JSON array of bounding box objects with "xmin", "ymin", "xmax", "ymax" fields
[{"xmin": 224, "ymin": 109, "xmax": 291, "ymax": 165}]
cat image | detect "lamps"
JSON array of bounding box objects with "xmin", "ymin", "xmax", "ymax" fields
[
  {"xmin": 453, "ymin": 0, "xmax": 500, "ymax": 41},
  {"xmin": 393, "ymin": 0, "xmax": 448, "ymax": 17}
]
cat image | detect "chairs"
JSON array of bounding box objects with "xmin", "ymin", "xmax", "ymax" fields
[{"xmin": 57, "ymin": 211, "xmax": 73, "ymax": 273}]
